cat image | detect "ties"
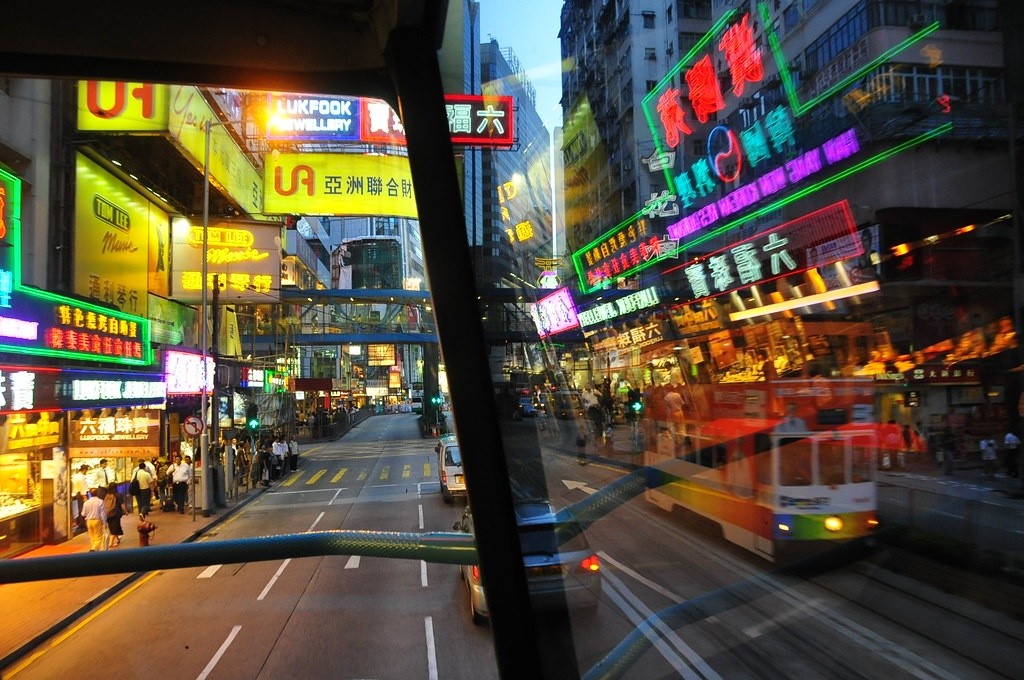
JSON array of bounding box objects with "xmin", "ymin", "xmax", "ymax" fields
[{"xmin": 104, "ymin": 470, "xmax": 108, "ymax": 485}]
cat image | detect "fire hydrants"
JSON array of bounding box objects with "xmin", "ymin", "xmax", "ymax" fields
[{"xmin": 137, "ymin": 520, "xmax": 158, "ymax": 547}]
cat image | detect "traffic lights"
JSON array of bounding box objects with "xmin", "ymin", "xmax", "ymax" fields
[
  {"xmin": 631, "ymin": 401, "xmax": 643, "ymax": 412},
  {"xmin": 246, "ymin": 402, "xmax": 258, "ymax": 430},
  {"xmin": 217, "ymin": 365, "xmax": 230, "ymax": 390},
  {"xmin": 430, "ymin": 395, "xmax": 441, "ymax": 406}
]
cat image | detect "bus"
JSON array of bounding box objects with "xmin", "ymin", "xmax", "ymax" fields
[
  {"xmin": 553, "ymin": 390, "xmax": 586, "ymax": 419},
  {"xmin": 411, "ymin": 382, "xmax": 445, "ymax": 414},
  {"xmin": 638, "ymin": 313, "xmax": 882, "ymax": 568}
]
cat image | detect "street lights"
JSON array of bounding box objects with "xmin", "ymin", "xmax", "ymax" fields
[{"xmin": 201, "ymin": 111, "xmax": 289, "ymax": 519}]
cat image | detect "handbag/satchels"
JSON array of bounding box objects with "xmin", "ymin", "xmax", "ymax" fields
[{"xmin": 262, "ymin": 469, "xmax": 269, "ymax": 480}]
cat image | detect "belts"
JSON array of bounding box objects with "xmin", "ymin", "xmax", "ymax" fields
[{"xmin": 173, "ymin": 482, "xmax": 186, "ymax": 484}]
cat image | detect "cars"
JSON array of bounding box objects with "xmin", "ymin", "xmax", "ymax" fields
[
  {"xmin": 521, "ymin": 402, "xmax": 535, "ymax": 416},
  {"xmin": 451, "ymin": 496, "xmax": 603, "ymax": 626}
]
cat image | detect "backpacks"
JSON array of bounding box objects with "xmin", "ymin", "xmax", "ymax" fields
[
  {"xmin": 129, "ymin": 472, "xmax": 140, "ymax": 495},
  {"xmin": 103, "ymin": 494, "xmax": 117, "ymax": 518}
]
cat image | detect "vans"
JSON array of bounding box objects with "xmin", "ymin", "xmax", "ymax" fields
[{"xmin": 435, "ymin": 434, "xmax": 469, "ymax": 504}]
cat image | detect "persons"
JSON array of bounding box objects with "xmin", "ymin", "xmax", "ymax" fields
[
  {"xmin": 72, "ymin": 433, "xmax": 299, "ymax": 554},
  {"xmin": 580, "ymin": 359, "xmax": 690, "ymax": 459},
  {"xmin": 877, "ymin": 418, "xmax": 1023, "ymax": 486},
  {"xmin": 299, "ymin": 405, "xmax": 360, "ymax": 436},
  {"xmin": 238, "ymin": 309, "xmax": 425, "ymax": 334},
  {"xmin": 750, "ymin": 349, "xmax": 776, "ymax": 383},
  {"xmin": 772, "ymin": 400, "xmax": 807, "ymax": 433}
]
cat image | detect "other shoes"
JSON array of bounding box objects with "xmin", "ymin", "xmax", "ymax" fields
[
  {"xmin": 116, "ymin": 536, "xmax": 122, "ymax": 543},
  {"xmin": 109, "ymin": 543, "xmax": 118, "ymax": 547},
  {"xmin": 140, "ymin": 513, "xmax": 145, "ymax": 522},
  {"xmin": 156, "ymin": 496, "xmax": 159, "ymax": 500}
]
